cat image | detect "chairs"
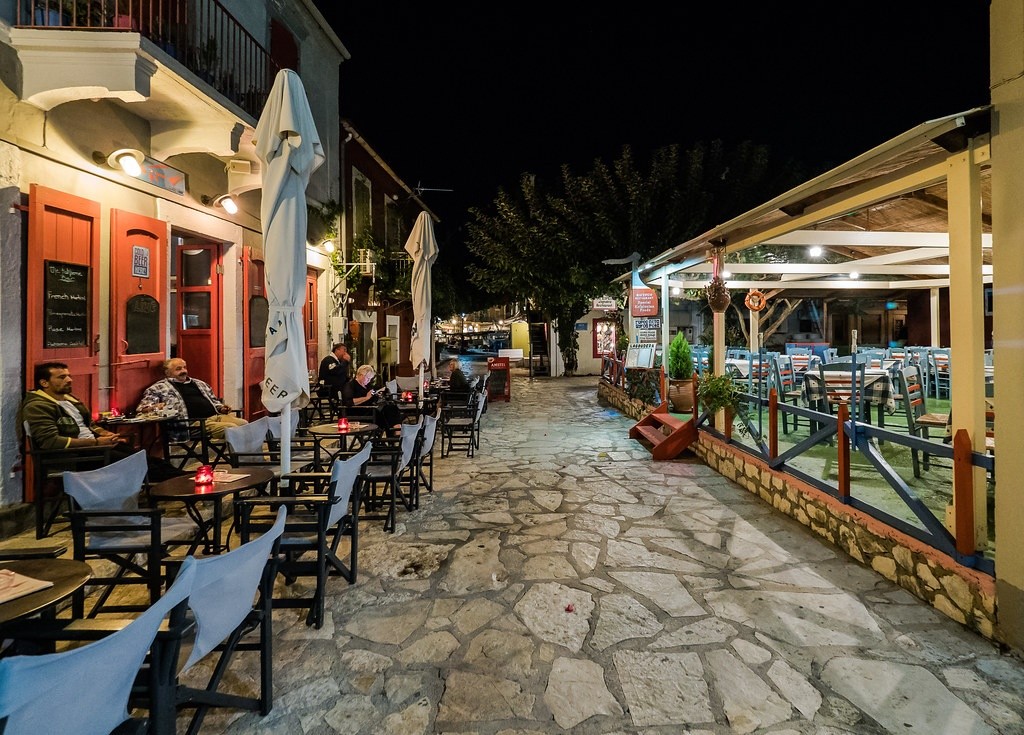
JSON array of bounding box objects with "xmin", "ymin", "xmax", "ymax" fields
[
  {"xmin": 687, "ymin": 343, "xmax": 996, "ymax": 486},
  {"xmin": 0, "ymin": 369, "xmax": 494, "ymax": 735}
]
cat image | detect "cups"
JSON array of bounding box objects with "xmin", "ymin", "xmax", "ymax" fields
[
  {"xmin": 348, "ymin": 422, "xmax": 360, "ymax": 429},
  {"xmin": 213, "ymin": 469, "xmax": 228, "ymax": 481}
]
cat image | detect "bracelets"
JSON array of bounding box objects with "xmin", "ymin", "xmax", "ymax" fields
[{"xmin": 95, "ymin": 438, "xmax": 98, "ymax": 445}]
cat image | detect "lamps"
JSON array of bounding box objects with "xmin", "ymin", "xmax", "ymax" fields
[
  {"xmin": 309, "ymin": 232, "xmax": 335, "ymax": 253},
  {"xmin": 200, "ymin": 192, "xmax": 238, "ymax": 215},
  {"xmin": 92, "ymin": 148, "xmax": 144, "ymax": 178}
]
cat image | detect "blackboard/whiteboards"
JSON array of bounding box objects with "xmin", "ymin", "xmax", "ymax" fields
[
  {"xmin": 126, "ymin": 295, "xmax": 160, "ymax": 354},
  {"xmin": 44, "ymin": 259, "xmax": 91, "ymax": 349}
]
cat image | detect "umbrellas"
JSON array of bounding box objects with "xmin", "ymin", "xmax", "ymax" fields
[
  {"xmin": 252, "ymin": 67, "xmax": 326, "ymax": 482},
  {"xmin": 404, "ymin": 211, "xmax": 439, "ymax": 424}
]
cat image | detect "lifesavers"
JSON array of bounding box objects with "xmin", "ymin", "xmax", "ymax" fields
[{"xmin": 745, "ymin": 290, "xmax": 766, "ymax": 311}]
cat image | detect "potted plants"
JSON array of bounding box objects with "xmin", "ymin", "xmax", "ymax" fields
[{"xmin": 667, "ymin": 329, "xmax": 697, "ymax": 413}]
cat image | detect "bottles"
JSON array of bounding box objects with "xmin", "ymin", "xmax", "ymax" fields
[
  {"xmin": 338, "ymin": 418, "xmax": 349, "ymax": 429},
  {"xmin": 195, "ymin": 465, "xmax": 213, "ymax": 485}
]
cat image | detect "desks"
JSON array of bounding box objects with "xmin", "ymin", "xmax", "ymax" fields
[
  {"xmin": 147, "ymin": 465, "xmax": 273, "ymax": 556},
  {"xmin": 702, "ymin": 359, "xmax": 769, "ymax": 389},
  {"xmin": 92, "ymin": 413, "xmax": 182, "ymax": 465},
  {"xmin": 1, "ymin": 557, "xmax": 93, "ymax": 658},
  {"xmin": 308, "ymin": 422, "xmax": 378, "ymax": 460},
  {"xmin": 800, "ymin": 368, "xmax": 894, "ymax": 448}
]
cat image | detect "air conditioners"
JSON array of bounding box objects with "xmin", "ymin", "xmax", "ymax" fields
[{"xmin": 355, "ymin": 248, "xmax": 376, "ymax": 273}]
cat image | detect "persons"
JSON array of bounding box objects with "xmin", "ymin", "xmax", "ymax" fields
[
  {"xmin": 316, "ymin": 343, "xmax": 471, "ymax": 447},
  {"xmin": 136, "ymin": 358, "xmax": 248, "ymax": 438},
  {"xmin": 22, "ymin": 362, "xmax": 215, "ymax": 506}
]
